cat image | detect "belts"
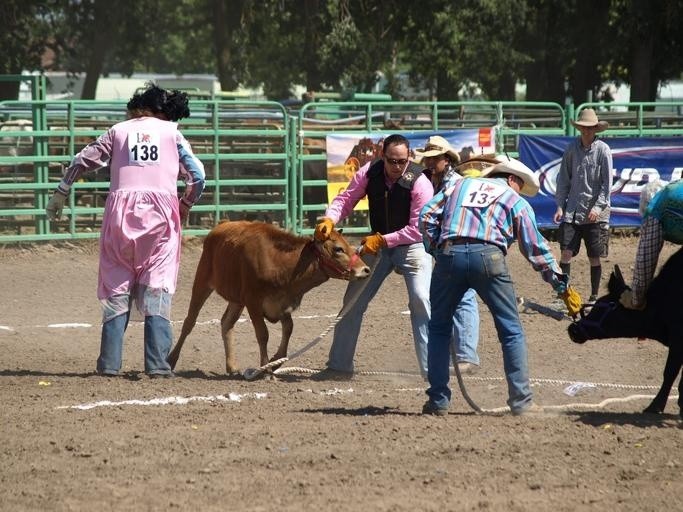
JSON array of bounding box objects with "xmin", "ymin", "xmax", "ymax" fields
[{"xmin": 446, "ymin": 238, "xmax": 484, "ymax": 245}]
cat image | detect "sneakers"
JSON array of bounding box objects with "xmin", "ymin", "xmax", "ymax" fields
[
  {"xmin": 449, "ymin": 362, "xmax": 478, "ymax": 376},
  {"xmin": 421, "ymin": 401, "xmax": 448, "ymax": 416},
  {"xmin": 514, "ymin": 400, "xmax": 544, "ymax": 416},
  {"xmin": 588, "ymin": 295, "xmax": 599, "ymax": 303},
  {"xmin": 311, "ymin": 368, "xmax": 353, "ymax": 381}
]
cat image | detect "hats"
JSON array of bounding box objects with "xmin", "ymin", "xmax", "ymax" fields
[
  {"xmin": 571, "ymin": 107, "xmax": 608, "ymax": 132},
  {"xmin": 413, "ymin": 136, "xmax": 461, "ymax": 164},
  {"xmin": 478, "ymin": 159, "xmax": 540, "ymax": 198}
]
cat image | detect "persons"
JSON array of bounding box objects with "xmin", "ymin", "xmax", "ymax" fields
[
  {"xmin": 308, "ymin": 134, "xmax": 437, "ymax": 382},
  {"xmin": 419, "ymin": 154, "xmax": 581, "ymax": 416},
  {"xmin": 552, "ymin": 108, "xmax": 614, "ymax": 308},
  {"xmin": 619, "ymin": 177, "xmax": 683, "ymax": 311},
  {"xmin": 414, "ymin": 135, "xmax": 481, "ymax": 377},
  {"xmin": 45, "ymin": 85, "xmax": 207, "ymax": 378}
]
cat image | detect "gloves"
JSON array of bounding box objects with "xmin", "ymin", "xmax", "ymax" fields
[
  {"xmin": 313, "ymin": 217, "xmax": 335, "ymax": 243},
  {"xmin": 556, "ymin": 284, "xmax": 584, "ymax": 319},
  {"xmin": 361, "ymin": 232, "xmax": 388, "ymax": 257},
  {"xmin": 618, "ymin": 289, "xmax": 647, "ymax": 311}
]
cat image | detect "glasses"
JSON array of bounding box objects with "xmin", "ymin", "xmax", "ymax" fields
[{"xmin": 382, "ymin": 153, "xmax": 409, "ymax": 165}]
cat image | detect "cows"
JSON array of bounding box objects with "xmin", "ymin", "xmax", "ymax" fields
[
  {"xmin": 167, "ymin": 219, "xmax": 372, "ymax": 382},
  {"xmin": 567, "ymin": 245, "xmax": 683, "ymax": 418}
]
